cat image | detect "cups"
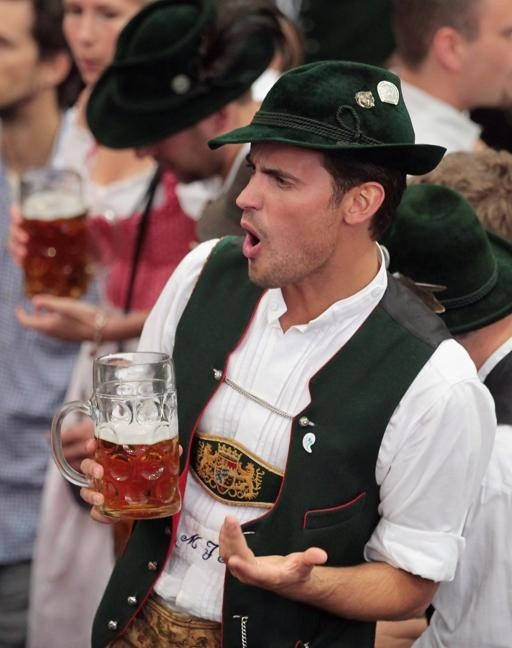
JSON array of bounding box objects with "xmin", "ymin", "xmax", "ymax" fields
[
  {"xmin": 20, "ymin": 169, "xmax": 87, "ymax": 298},
  {"xmin": 50, "ymin": 351, "xmax": 182, "ymax": 519}
]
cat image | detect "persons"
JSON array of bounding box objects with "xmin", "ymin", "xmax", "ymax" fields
[{"xmin": 2, "ymin": 2, "xmax": 510, "ymax": 647}]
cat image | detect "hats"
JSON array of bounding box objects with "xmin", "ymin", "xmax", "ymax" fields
[
  {"xmin": 383, "ymin": 181, "xmax": 512, "ymax": 332},
  {"xmin": 207, "ymin": 60, "xmax": 447, "ymax": 175},
  {"xmin": 85, "ymin": 0, "xmax": 276, "ymax": 149}
]
